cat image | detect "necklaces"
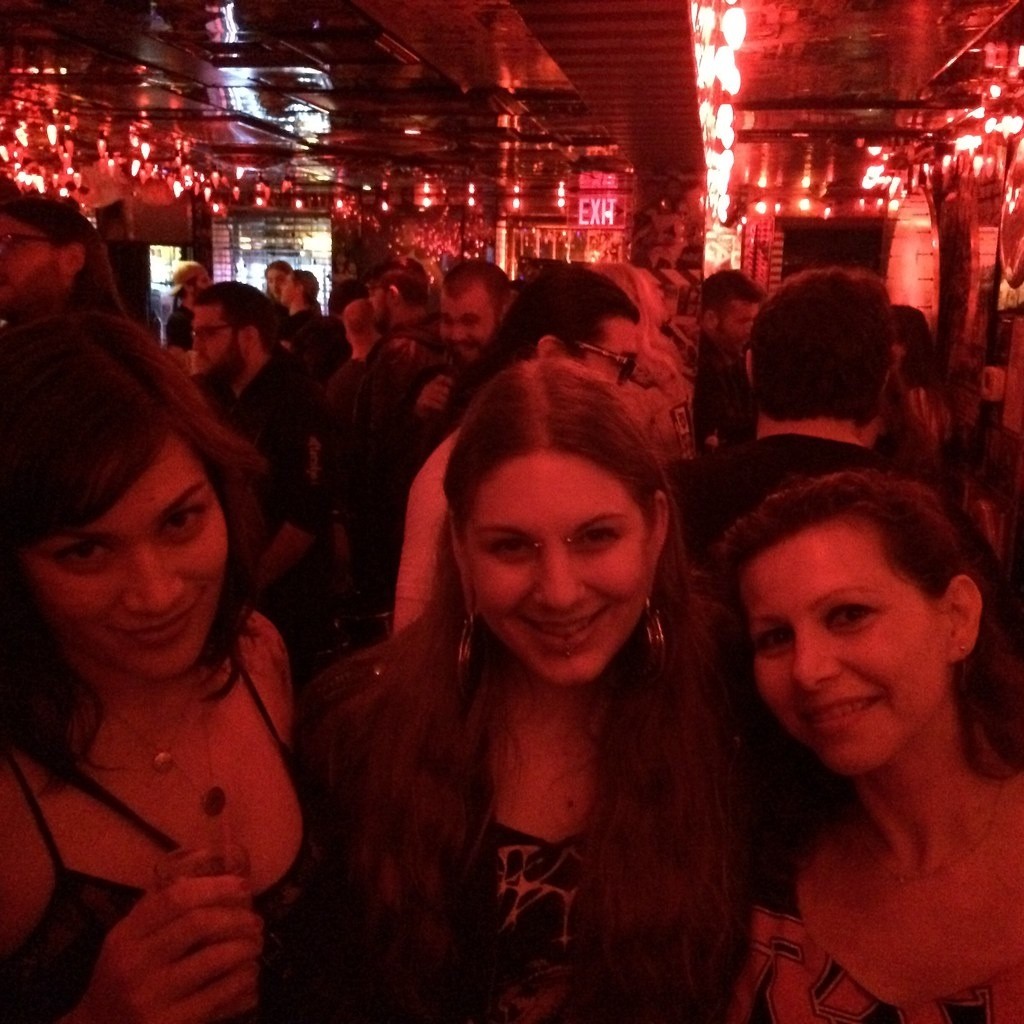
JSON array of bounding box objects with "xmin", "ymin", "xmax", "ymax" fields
[
  {"xmin": 129, "ymin": 685, "xmax": 228, "ymax": 816},
  {"xmin": 847, "ymin": 817, "xmax": 990, "ymax": 882}
]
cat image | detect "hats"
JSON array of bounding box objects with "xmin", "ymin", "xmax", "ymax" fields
[{"xmin": 168, "ymin": 260, "xmax": 203, "ymax": 296}]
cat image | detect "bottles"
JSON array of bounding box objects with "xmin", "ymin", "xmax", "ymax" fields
[{"xmin": 415, "ymin": 352, "xmax": 461, "ymax": 440}]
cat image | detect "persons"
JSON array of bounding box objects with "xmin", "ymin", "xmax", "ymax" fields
[{"xmin": 0, "ymin": 196, "xmax": 1023, "ymax": 1024}]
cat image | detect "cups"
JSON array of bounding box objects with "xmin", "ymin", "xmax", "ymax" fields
[{"xmin": 146, "ymin": 841, "xmax": 259, "ymax": 1020}]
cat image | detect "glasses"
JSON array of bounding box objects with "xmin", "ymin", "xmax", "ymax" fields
[
  {"xmin": 531, "ymin": 334, "xmax": 637, "ymax": 386},
  {"xmin": 191, "ymin": 324, "xmax": 233, "ymax": 345},
  {"xmin": 0, "ymin": 233, "xmax": 63, "ymax": 255}
]
